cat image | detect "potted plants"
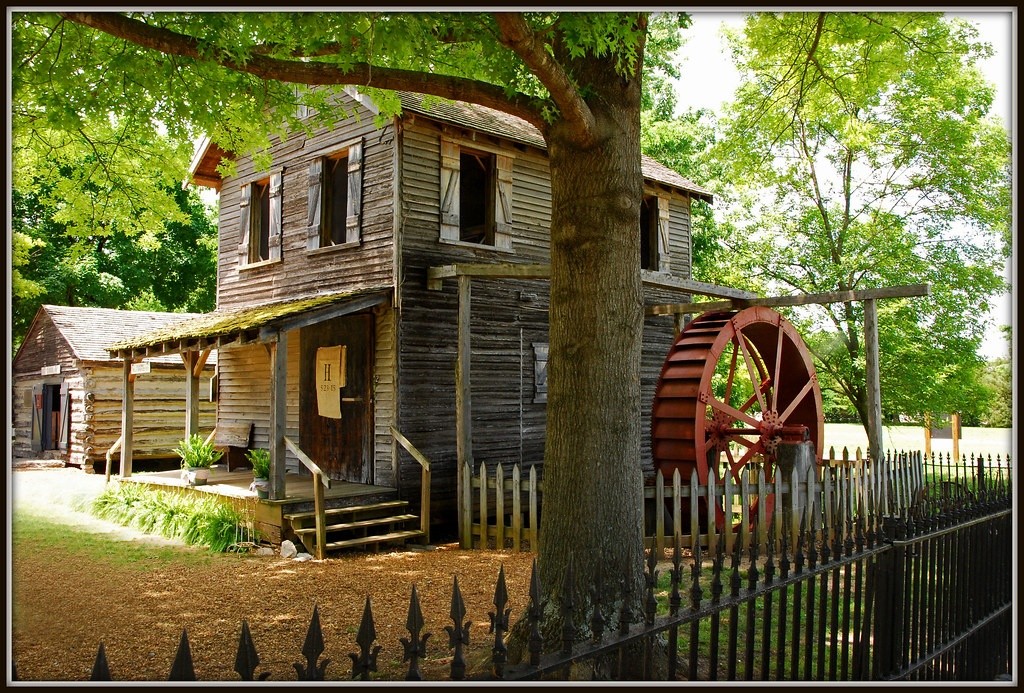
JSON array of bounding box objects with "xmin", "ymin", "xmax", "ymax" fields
[
  {"xmin": 172, "ymin": 434, "xmax": 225, "ymax": 486},
  {"xmin": 244, "ymin": 447, "xmax": 270, "ymax": 498}
]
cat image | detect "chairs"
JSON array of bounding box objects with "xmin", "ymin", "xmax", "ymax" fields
[{"xmin": 211, "ymin": 420, "xmax": 253, "ymax": 473}]
